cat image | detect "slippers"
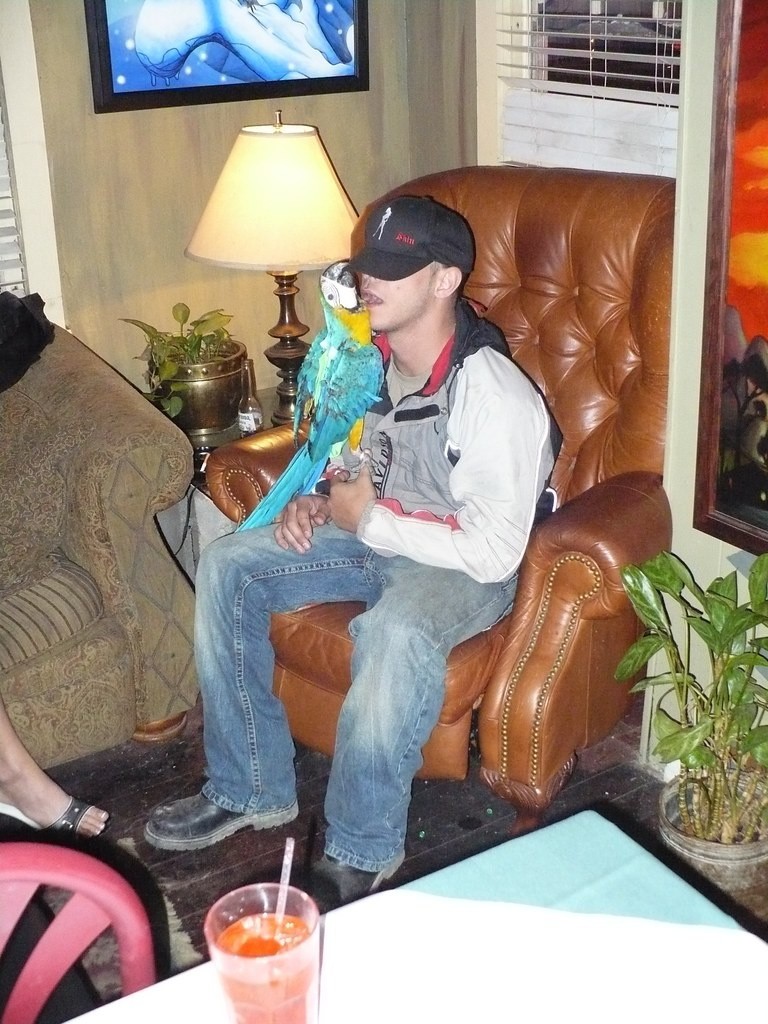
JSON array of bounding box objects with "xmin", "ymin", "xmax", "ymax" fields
[{"xmin": 0, "ymin": 795, "xmax": 114, "ymax": 839}]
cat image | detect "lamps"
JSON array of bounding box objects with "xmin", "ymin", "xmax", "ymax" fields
[{"xmin": 184, "ymin": 110, "xmax": 360, "ymax": 425}]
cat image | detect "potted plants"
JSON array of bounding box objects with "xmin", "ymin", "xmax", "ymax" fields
[
  {"xmin": 615, "ymin": 550, "xmax": 768, "ymax": 943},
  {"xmin": 119, "ymin": 302, "xmax": 248, "ymax": 448}
]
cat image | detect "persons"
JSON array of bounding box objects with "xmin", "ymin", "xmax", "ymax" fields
[
  {"xmin": 0, "ymin": 696, "xmax": 115, "ymax": 839},
  {"xmin": 142, "ymin": 194, "xmax": 563, "ymax": 910}
]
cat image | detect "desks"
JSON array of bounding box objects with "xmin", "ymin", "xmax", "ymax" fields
[{"xmin": 67, "ymin": 811, "xmax": 768, "ymax": 1024}]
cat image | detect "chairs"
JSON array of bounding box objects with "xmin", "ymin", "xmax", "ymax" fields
[{"xmin": 0, "ymin": 846, "xmax": 154, "ymax": 1024}]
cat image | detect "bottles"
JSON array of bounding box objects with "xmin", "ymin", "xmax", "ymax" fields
[{"xmin": 237, "ymin": 360, "xmax": 265, "ymax": 438}]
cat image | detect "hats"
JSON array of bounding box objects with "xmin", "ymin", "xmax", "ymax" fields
[{"xmin": 347, "ymin": 195, "xmax": 474, "ymax": 282}]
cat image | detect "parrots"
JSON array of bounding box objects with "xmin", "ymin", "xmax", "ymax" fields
[{"xmin": 231, "ymin": 260, "xmax": 384, "ymax": 533}]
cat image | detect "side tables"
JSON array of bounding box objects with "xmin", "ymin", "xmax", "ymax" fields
[{"xmin": 155, "ymin": 386, "xmax": 279, "ymax": 594}]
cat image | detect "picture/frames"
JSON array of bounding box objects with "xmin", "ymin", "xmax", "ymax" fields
[{"xmin": 693, "ymin": 0, "xmax": 768, "ymax": 558}]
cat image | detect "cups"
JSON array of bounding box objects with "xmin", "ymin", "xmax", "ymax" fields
[{"xmin": 204, "ymin": 883, "xmax": 320, "ymax": 1024}]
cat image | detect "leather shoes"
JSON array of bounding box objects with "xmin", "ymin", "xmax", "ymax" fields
[
  {"xmin": 307, "ymin": 849, "xmax": 408, "ymax": 904},
  {"xmin": 143, "ymin": 790, "xmax": 301, "ymax": 851}
]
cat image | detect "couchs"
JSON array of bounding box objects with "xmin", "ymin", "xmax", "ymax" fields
[
  {"xmin": 205, "ymin": 166, "xmax": 676, "ymax": 840},
  {"xmin": 0, "ymin": 322, "xmax": 200, "ymax": 770}
]
